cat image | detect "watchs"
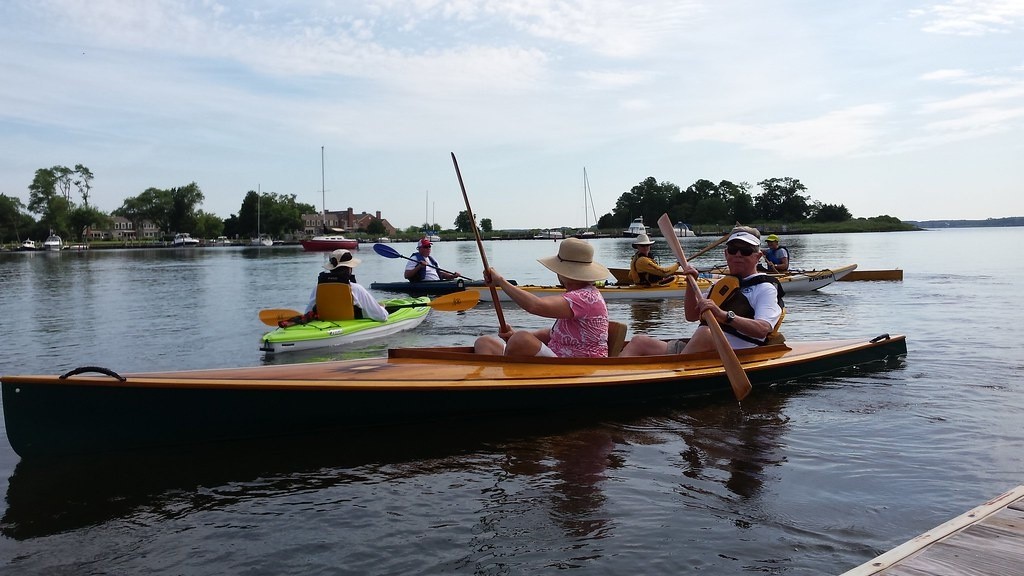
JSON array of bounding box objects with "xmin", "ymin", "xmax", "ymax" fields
[{"xmin": 725, "ymin": 311, "xmax": 735, "ymax": 325}]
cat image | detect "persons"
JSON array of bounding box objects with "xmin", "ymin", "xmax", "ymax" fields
[
  {"xmin": 629, "ymin": 234, "xmax": 681, "ymax": 286},
  {"xmin": 474, "ymin": 238, "xmax": 609, "ymax": 357},
  {"xmin": 757, "ymin": 234, "xmax": 790, "ymax": 272},
  {"xmin": 620, "ymin": 227, "xmax": 785, "ymax": 356},
  {"xmin": 405, "ymin": 239, "xmax": 460, "ymax": 281},
  {"xmin": 305, "ymin": 249, "xmax": 388, "ymax": 321}
]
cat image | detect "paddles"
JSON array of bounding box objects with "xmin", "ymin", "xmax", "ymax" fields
[
  {"xmin": 657, "ymin": 213, "xmax": 753, "ymax": 403},
  {"xmin": 678, "ymin": 231, "xmax": 731, "ymax": 266},
  {"xmin": 373, "ymin": 243, "xmax": 474, "ymax": 281},
  {"xmin": 258, "ymin": 290, "xmax": 480, "ymax": 328},
  {"xmin": 735, "ymin": 220, "xmax": 781, "ymax": 274},
  {"xmin": 450, "ymin": 150, "xmax": 508, "ymax": 339}
]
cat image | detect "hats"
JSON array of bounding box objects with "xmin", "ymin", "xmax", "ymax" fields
[
  {"xmin": 726, "ymin": 231, "xmax": 761, "ymax": 246},
  {"xmin": 765, "ymin": 234, "xmax": 778, "ymax": 242},
  {"xmin": 632, "ymin": 234, "xmax": 655, "ymax": 249},
  {"xmin": 418, "ymin": 238, "xmax": 433, "ymax": 247},
  {"xmin": 322, "ymin": 249, "xmax": 361, "ymax": 270},
  {"xmin": 536, "ymin": 237, "xmax": 610, "ymax": 281}
]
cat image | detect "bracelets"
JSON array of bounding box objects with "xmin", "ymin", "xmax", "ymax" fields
[{"xmin": 775, "ymin": 264, "xmax": 777, "ymax": 268}]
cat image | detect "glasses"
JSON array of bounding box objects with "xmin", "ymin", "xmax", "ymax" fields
[
  {"xmin": 422, "ymin": 245, "xmax": 432, "ymax": 248},
  {"xmin": 640, "ymin": 244, "xmax": 651, "ymax": 247},
  {"xmin": 727, "ymin": 243, "xmax": 756, "ymax": 256}
]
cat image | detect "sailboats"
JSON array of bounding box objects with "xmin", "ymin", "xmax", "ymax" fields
[{"xmin": 570, "ymin": 166, "xmax": 611, "ymax": 239}]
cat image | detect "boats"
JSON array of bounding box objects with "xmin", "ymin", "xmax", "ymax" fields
[
  {"xmin": 21, "ymin": 238, "xmax": 35, "ymax": 249},
  {"xmin": 622, "ymin": 216, "xmax": 652, "ymax": 238},
  {"xmin": 209, "ymin": 236, "xmax": 231, "ymax": 247},
  {"xmin": 262, "ymin": 297, "xmax": 432, "ymax": 355},
  {"xmin": 251, "ymin": 234, "xmax": 274, "ymax": 247},
  {"xmin": 426, "ymin": 234, "xmax": 441, "ymax": 242},
  {"xmin": 171, "ymin": 232, "xmax": 199, "ymax": 247},
  {"xmin": 713, "ymin": 264, "xmax": 859, "ymax": 292},
  {"xmin": 44, "ymin": 234, "xmax": 63, "ymax": 251},
  {"xmin": 299, "ymin": 235, "xmax": 358, "ymax": 251},
  {"xmin": 465, "ymin": 275, "xmax": 713, "ymax": 302},
  {"xmin": 673, "ymin": 222, "xmax": 696, "ymax": 237},
  {"xmin": 369, "ymin": 279, "xmax": 518, "ymax": 297},
  {"xmin": 0, "ymin": 334, "xmax": 907, "ymax": 461}
]
